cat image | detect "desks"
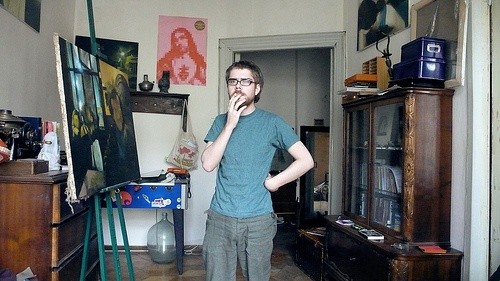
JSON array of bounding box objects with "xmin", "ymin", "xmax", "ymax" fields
[{"xmin": 100, "ymin": 182, "xmax": 189, "ymax": 275}]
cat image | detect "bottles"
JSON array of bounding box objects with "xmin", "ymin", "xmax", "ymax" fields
[
  {"xmin": 158, "ymin": 69, "xmax": 170, "ymax": 92},
  {"xmin": 138, "ymin": 73, "xmax": 154, "ymax": 91},
  {"xmin": 146, "ymin": 213, "xmax": 176, "ymax": 263}
]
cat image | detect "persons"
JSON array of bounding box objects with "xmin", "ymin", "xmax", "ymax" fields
[
  {"xmin": 201, "ymin": 58, "xmax": 316, "ymax": 281},
  {"xmin": 376, "ymin": 117, "xmax": 389, "ymax": 135}
]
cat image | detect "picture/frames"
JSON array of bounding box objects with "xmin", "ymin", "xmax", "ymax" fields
[{"xmin": 357, "ymin": 0, "xmax": 409, "ymax": 52}]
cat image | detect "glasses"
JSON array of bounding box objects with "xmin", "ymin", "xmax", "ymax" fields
[{"xmin": 227, "ymin": 78, "xmax": 259, "ymax": 86}]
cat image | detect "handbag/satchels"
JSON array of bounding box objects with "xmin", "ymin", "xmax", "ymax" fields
[{"xmin": 166, "ymin": 100, "xmax": 199, "ymax": 171}]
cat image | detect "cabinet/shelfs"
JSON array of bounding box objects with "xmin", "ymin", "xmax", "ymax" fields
[
  {"xmin": 320, "ymin": 88, "xmax": 464, "ymax": 281},
  {"xmin": 0, "ymin": 169, "xmax": 98, "ymax": 281}
]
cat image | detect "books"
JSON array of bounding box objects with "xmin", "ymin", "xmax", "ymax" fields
[{"xmin": 307, "ymin": 161, "xmax": 403, "ymax": 240}]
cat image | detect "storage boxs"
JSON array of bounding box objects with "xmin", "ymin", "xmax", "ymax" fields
[{"xmin": 393, "ymin": 36, "xmax": 447, "ymax": 81}]
